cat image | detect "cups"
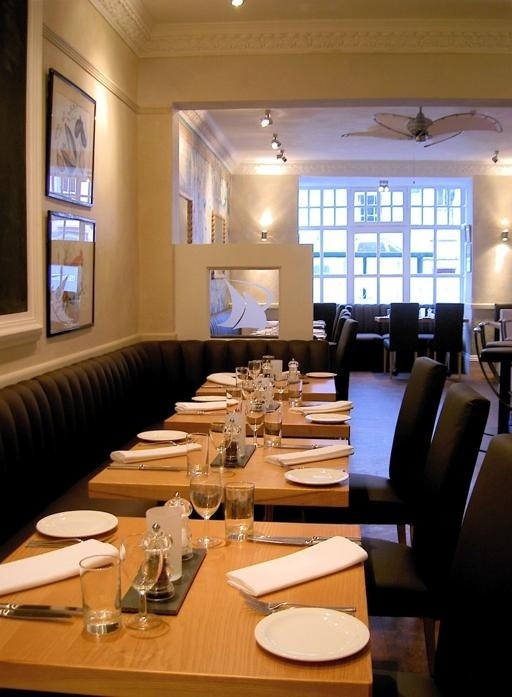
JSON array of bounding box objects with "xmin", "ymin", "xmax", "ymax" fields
[
  {"xmin": 224, "ymin": 414, "xmax": 246, "ymax": 456},
  {"xmin": 263, "ymin": 410, "xmax": 282, "ymax": 449},
  {"xmin": 261, "ymin": 376, "xmax": 273, "ymax": 404},
  {"xmin": 288, "ymin": 379, "xmax": 303, "ymax": 407},
  {"xmin": 186, "ymin": 433, "xmax": 209, "ymax": 480},
  {"xmin": 270, "ymin": 360, "xmax": 282, "ymax": 376},
  {"xmin": 248, "ymin": 361, "xmax": 261, "ymax": 379},
  {"xmin": 189, "ymin": 466, "xmax": 223, "ymax": 549},
  {"xmin": 146, "ymin": 507, "xmax": 182, "ymax": 582},
  {"xmin": 79, "ymin": 555, "xmax": 125, "ymax": 643},
  {"xmin": 224, "ymin": 482, "xmax": 254, "ymax": 542},
  {"xmin": 226, "ymin": 387, "xmax": 242, "ymax": 414},
  {"xmin": 210, "ymin": 426, "xmax": 232, "ymax": 473},
  {"xmin": 236, "ymin": 367, "xmax": 248, "ymax": 390},
  {"xmin": 121, "ymin": 534, "xmax": 162, "ymax": 631},
  {"xmin": 246, "ymin": 402, "xmax": 266, "ymax": 448}
]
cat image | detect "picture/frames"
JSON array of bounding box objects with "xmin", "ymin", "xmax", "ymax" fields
[
  {"xmin": 45, "ymin": 210, "xmax": 96, "ymax": 337},
  {"xmin": 212, "ymin": 210, "xmax": 226, "ymax": 279},
  {"xmin": 178, "ymin": 191, "xmax": 193, "ymax": 244},
  {"xmin": 1, "ymin": 1, "xmax": 44, "ymax": 341},
  {"xmin": 42, "ymin": 67, "xmax": 96, "ymax": 208}
]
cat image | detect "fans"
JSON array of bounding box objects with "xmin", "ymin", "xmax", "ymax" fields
[{"xmin": 340, "ymin": 106, "xmax": 504, "ymax": 148}]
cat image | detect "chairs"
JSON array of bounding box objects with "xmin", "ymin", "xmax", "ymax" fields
[
  {"xmin": 333, "ymin": 316, "xmax": 348, "ymax": 342},
  {"xmin": 350, "ymin": 357, "xmax": 448, "ymax": 545},
  {"xmin": 332, "ymin": 305, "xmax": 345, "ymax": 340},
  {"xmin": 361, "ymin": 382, "xmax": 492, "ymax": 674},
  {"xmin": 373, "ymin": 434, "xmax": 512, "ymax": 696},
  {"xmin": 328, "ymin": 319, "xmax": 358, "ymax": 401},
  {"xmin": 426, "ymin": 303, "xmax": 465, "ymax": 379},
  {"xmin": 344, "ymin": 312, "xmax": 351, "ymax": 317},
  {"xmin": 334, "ymin": 309, "xmax": 348, "ymax": 342},
  {"xmin": 383, "ymin": 303, "xmax": 420, "ymax": 379}
]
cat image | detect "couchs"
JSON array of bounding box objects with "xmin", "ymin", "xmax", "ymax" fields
[
  {"xmin": 494, "ymin": 303, "xmax": 512, "ymax": 341},
  {"xmin": 346, "ymin": 303, "xmax": 463, "ymax": 371},
  {"xmin": 1, "ymin": 339, "xmax": 328, "ymax": 542}
]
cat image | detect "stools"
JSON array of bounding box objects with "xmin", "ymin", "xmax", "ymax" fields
[
  {"xmin": 473, "ymin": 327, "xmax": 512, "ymax": 433},
  {"xmin": 479, "ymin": 321, "xmax": 512, "ymax": 397}
]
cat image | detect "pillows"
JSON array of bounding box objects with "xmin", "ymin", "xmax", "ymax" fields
[{"xmin": 499, "ymin": 309, "xmax": 512, "ymax": 340}]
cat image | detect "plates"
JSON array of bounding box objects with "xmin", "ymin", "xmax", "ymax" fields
[
  {"xmin": 36, "ymin": 510, "xmax": 118, "ymax": 537},
  {"xmin": 192, "ymin": 396, "xmax": 226, "ymax": 401},
  {"xmin": 284, "ymin": 467, "xmax": 349, "ymax": 485},
  {"xmin": 137, "ymin": 430, "xmax": 188, "ymax": 440},
  {"xmin": 306, "ymin": 372, "xmax": 336, "ymax": 377},
  {"xmin": 306, "ymin": 414, "xmax": 350, "ymax": 423},
  {"xmin": 254, "ymin": 608, "xmax": 371, "ymax": 662}
]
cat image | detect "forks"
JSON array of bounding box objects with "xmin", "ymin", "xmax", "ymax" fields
[
  {"xmin": 239, "ymin": 594, "xmax": 358, "ymax": 615},
  {"xmin": 25, "ymin": 535, "xmax": 119, "ymax": 547}
]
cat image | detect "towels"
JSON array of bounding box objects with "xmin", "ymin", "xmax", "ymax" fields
[
  {"xmin": 263, "ymin": 443, "xmax": 354, "ymax": 467},
  {"xmin": 224, "ymin": 535, "xmax": 369, "ymax": 597},
  {"xmin": 110, "ymin": 442, "xmax": 202, "ymax": 464},
  {"xmin": 206, "ymin": 372, "xmax": 243, "ymax": 386},
  {"xmin": 0, "ymin": 537, "xmax": 119, "ymax": 599},
  {"xmin": 173, "ymin": 399, "xmax": 237, "ymax": 416},
  {"xmin": 289, "ymin": 399, "xmax": 352, "ymax": 415}
]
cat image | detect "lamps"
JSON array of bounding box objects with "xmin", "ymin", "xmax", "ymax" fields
[
  {"xmin": 271, "ymin": 133, "xmax": 282, "ymax": 149},
  {"xmin": 260, "ymin": 110, "xmax": 273, "ymax": 128}
]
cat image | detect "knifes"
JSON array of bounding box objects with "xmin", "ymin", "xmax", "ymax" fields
[
  {"xmin": 0, "ymin": 608, "xmax": 73, "ymax": 620},
  {"xmin": 107, "ymin": 464, "xmax": 181, "ymax": 471},
  {"xmin": 0, "ymin": 602, "xmax": 85, "ymax": 613},
  {"xmin": 246, "ymin": 535, "xmax": 362, "ymax": 545},
  {"xmin": 282, "ymin": 443, "xmax": 332, "ymax": 448}
]
